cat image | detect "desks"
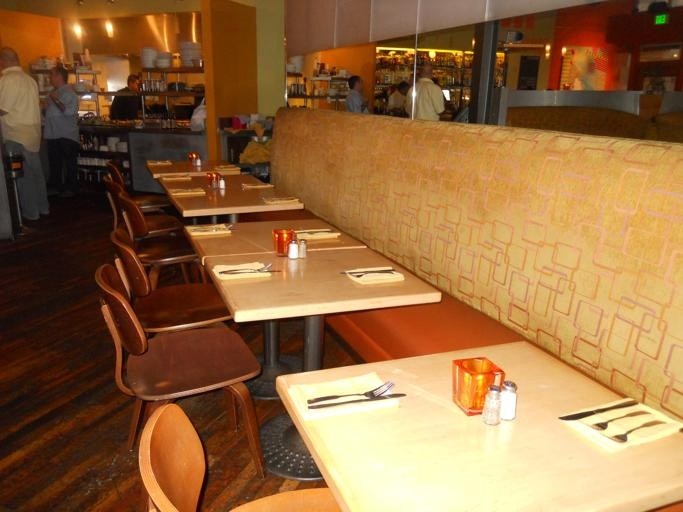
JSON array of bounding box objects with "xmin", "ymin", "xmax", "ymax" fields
[
  {"xmin": 147, "ymin": 159, "xmax": 241, "ymax": 180},
  {"xmin": 274, "ymin": 338, "xmax": 682, "ymax": 512},
  {"xmin": 203, "ymin": 247, "xmax": 443, "ymax": 481},
  {"xmin": 185, "ymin": 219, "xmax": 368, "ymax": 401},
  {"xmin": 159, "ymin": 175, "xmax": 305, "ymax": 224}
]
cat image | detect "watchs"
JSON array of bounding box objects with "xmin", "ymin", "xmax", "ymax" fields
[{"xmin": 54, "ymin": 100, "xmax": 59, "ymax": 105}]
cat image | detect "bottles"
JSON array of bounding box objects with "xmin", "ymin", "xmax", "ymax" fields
[
  {"xmin": 192, "ymin": 156, "xmax": 201, "ymax": 167},
  {"xmin": 212, "ymin": 178, "xmax": 218, "ymax": 189},
  {"xmin": 481, "ymin": 386, "xmax": 501, "ymax": 426},
  {"xmin": 146, "ymin": 111, "xmax": 176, "ymax": 129},
  {"xmin": 499, "ymin": 381, "xmax": 518, "ymax": 423},
  {"xmin": 219, "ymin": 178, "xmax": 225, "ymax": 189},
  {"xmin": 140, "ymin": 77, "xmax": 167, "ymax": 93},
  {"xmin": 287, "ymin": 240, "xmax": 297, "ymax": 259},
  {"xmin": 329, "ymin": 66, "xmax": 336, "ymax": 76},
  {"xmin": 297, "ymin": 238, "xmax": 308, "ymax": 259},
  {"xmin": 374, "ymin": 53, "xmax": 458, "ymax": 99},
  {"xmin": 315, "ymin": 62, "xmax": 320, "ymax": 77}
]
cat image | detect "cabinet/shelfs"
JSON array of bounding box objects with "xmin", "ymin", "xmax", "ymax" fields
[
  {"xmin": 375, "ymin": 46, "xmax": 505, "ymax": 122},
  {"xmin": 30, "ymin": 68, "xmax": 102, "ymax": 118},
  {"xmin": 140, "ymin": 67, "xmax": 205, "ymax": 121},
  {"xmin": 285, "ymin": 72, "xmax": 352, "ymax": 112},
  {"xmin": 78, "ymin": 125, "xmax": 133, "ymax": 183}
]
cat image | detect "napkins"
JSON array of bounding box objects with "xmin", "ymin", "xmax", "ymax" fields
[
  {"xmin": 287, "ymin": 372, "xmax": 401, "ymax": 424},
  {"xmin": 186, "ymin": 223, "xmax": 231, "ymax": 236},
  {"xmin": 560, "ymin": 395, "xmax": 682, "ymax": 454},
  {"xmin": 169, "ymin": 188, "xmax": 206, "ymax": 196},
  {"xmin": 291, "ymin": 228, "xmax": 342, "ymax": 242},
  {"xmin": 149, "ymin": 160, "xmax": 171, "ymax": 166},
  {"xmin": 346, "ymin": 265, "xmax": 405, "ymax": 285},
  {"xmin": 262, "ymin": 195, "xmax": 299, "ymax": 204},
  {"xmin": 242, "ymin": 181, "xmax": 274, "ymax": 190},
  {"xmin": 216, "ymin": 164, "xmax": 240, "ymax": 172},
  {"xmin": 212, "ymin": 261, "xmax": 272, "ymax": 279}
]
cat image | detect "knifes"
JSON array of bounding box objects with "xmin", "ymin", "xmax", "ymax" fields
[
  {"xmin": 191, "ymin": 224, "xmax": 238, "ymax": 233},
  {"xmin": 306, "ymin": 393, "xmax": 408, "ymax": 412},
  {"xmin": 339, "ymin": 268, "xmax": 396, "ymax": 275},
  {"xmin": 296, "ymin": 230, "xmax": 333, "ymax": 234},
  {"xmin": 219, "ymin": 269, "xmax": 282, "ymax": 274},
  {"xmin": 557, "ymin": 399, "xmax": 639, "ymax": 420}
]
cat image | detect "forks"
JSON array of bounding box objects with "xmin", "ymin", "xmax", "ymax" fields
[
  {"xmin": 604, "ymin": 420, "xmax": 665, "ymax": 444},
  {"xmin": 584, "ymin": 408, "xmax": 650, "ymax": 431},
  {"xmin": 218, "ymin": 263, "xmax": 273, "ymax": 272},
  {"xmin": 350, "ymin": 271, "xmax": 393, "ymax": 278},
  {"xmin": 305, "ymin": 380, "xmax": 393, "ymax": 404}
]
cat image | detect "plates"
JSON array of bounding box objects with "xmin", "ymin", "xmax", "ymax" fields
[
  {"xmin": 287, "ymin": 54, "xmax": 304, "ymax": 75},
  {"xmin": 141, "ymin": 42, "xmax": 201, "ymax": 68},
  {"xmin": 327, "ymin": 89, "xmax": 337, "ymax": 96},
  {"xmin": 99, "ymin": 137, "xmax": 128, "ymax": 153}
]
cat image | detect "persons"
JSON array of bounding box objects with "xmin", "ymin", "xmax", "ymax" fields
[
  {"xmin": 37, "ymin": 63, "xmax": 81, "ymax": 199},
  {"xmin": 343, "ymin": 75, "xmax": 374, "ymax": 116},
  {"xmin": 107, "ymin": 73, "xmax": 141, "ymax": 122},
  {"xmin": 386, "ymin": 80, "xmax": 413, "ymax": 120},
  {"xmin": 380, "ymin": 86, "xmax": 397, "ymax": 117},
  {"xmin": 405, "ymin": 64, "xmax": 446, "ymax": 122},
  {"xmin": 0, "ymin": 44, "xmax": 52, "ymax": 225}
]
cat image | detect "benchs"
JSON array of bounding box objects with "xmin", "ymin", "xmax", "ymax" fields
[{"xmin": 244, "ymin": 106, "xmax": 683, "ymax": 511}]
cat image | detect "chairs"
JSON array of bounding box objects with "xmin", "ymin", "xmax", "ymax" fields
[
  {"xmin": 121, "ymin": 191, "xmax": 181, "ymax": 244},
  {"xmin": 137, "ymin": 403, "xmax": 341, "ymax": 510},
  {"xmin": 94, "ymin": 264, "xmax": 268, "ymax": 481},
  {"xmin": 110, "ymin": 228, "xmax": 235, "ymax": 333},
  {"xmin": 105, "ymin": 163, "xmax": 179, "ymax": 226}
]
